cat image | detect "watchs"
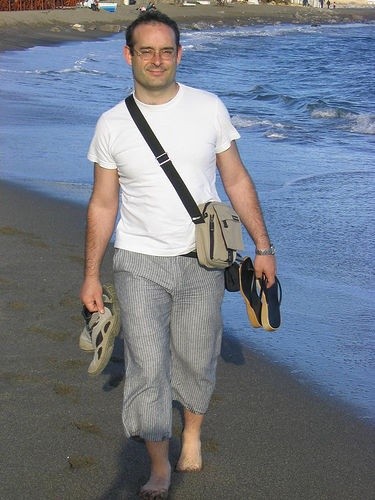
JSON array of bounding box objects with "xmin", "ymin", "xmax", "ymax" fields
[{"xmin": 254, "ymin": 243, "xmax": 276, "ymax": 256}]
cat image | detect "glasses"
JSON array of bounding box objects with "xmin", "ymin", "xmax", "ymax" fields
[{"xmin": 128, "ymin": 46, "xmax": 178, "ymax": 61}]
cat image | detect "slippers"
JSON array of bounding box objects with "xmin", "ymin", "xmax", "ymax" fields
[
  {"xmin": 255, "ymin": 270, "xmax": 282, "ymax": 332},
  {"xmin": 238, "ymin": 256, "xmax": 261, "ymax": 328}
]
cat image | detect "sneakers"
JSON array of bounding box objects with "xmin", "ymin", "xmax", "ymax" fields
[
  {"xmin": 86, "ymin": 298, "xmax": 121, "ymax": 376},
  {"xmin": 79, "ymin": 283, "xmax": 115, "ymax": 353}
]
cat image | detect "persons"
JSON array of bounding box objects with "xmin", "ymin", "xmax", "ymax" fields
[
  {"xmin": 333, "ymin": 2, "xmax": 336, "ymax": 8},
  {"xmin": 81, "ymin": 8, "xmax": 278, "ymax": 499},
  {"xmin": 139, "ymin": 2, "xmax": 157, "ymax": 11},
  {"xmin": 327, "ymin": 0, "xmax": 331, "ymax": 8}
]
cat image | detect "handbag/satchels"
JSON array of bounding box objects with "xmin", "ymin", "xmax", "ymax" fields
[{"xmin": 195, "ymin": 202, "xmax": 244, "ymax": 270}]
